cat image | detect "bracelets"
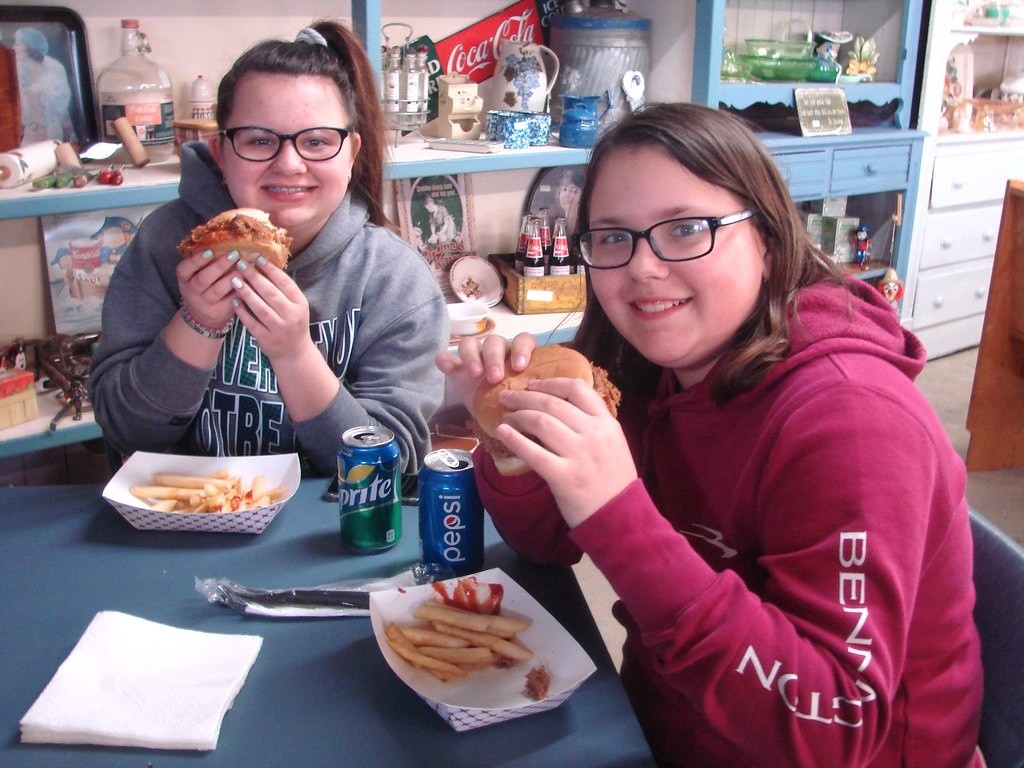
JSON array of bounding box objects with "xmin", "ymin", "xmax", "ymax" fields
[{"xmin": 181, "ymin": 308, "xmax": 235, "ymax": 339}]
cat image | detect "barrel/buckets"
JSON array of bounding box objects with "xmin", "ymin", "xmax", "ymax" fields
[{"xmin": 551, "ymin": 0, "xmax": 653, "ymax": 131}]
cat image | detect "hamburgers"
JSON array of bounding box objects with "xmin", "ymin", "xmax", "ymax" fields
[
  {"xmin": 463, "ymin": 343, "xmax": 621, "ymax": 476},
  {"xmin": 177, "ymin": 207, "xmax": 293, "ymax": 272}
]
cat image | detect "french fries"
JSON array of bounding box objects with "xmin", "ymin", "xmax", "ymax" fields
[
  {"xmin": 383, "ymin": 602, "xmax": 535, "ymax": 683},
  {"xmin": 130, "ymin": 469, "xmax": 286, "ymax": 515}
]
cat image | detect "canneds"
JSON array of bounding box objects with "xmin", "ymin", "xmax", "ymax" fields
[
  {"xmin": 417, "ymin": 449, "xmax": 485, "ymax": 577},
  {"xmin": 337, "ymin": 425, "xmax": 403, "ymax": 550}
]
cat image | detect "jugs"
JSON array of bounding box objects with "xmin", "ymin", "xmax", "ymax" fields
[{"xmin": 493, "ymin": 39, "xmax": 560, "ymax": 111}]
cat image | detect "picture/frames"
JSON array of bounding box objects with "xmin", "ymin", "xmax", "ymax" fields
[{"xmin": 0, "ymin": 5, "xmax": 100, "ymax": 166}]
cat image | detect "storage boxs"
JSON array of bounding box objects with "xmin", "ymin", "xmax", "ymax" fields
[
  {"xmin": 369, "ymin": 568, "xmax": 597, "ymax": 732},
  {"xmin": 0, "ymin": 368, "xmax": 38, "ymax": 430},
  {"xmin": 102, "ymin": 450, "xmax": 301, "ymax": 535},
  {"xmin": 486, "ymin": 253, "xmax": 587, "ymax": 314},
  {"xmin": 797, "ymin": 196, "xmax": 860, "ymax": 263}
]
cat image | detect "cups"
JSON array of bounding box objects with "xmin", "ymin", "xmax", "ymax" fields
[
  {"xmin": 486, "ymin": 111, "xmax": 552, "ymax": 149},
  {"xmin": 560, "ymin": 95, "xmax": 601, "ymax": 118}
]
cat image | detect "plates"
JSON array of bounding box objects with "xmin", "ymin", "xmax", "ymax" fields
[
  {"xmin": 450, "ymin": 318, "xmax": 496, "ymax": 344},
  {"xmin": 450, "ymin": 256, "xmax": 505, "ymax": 308}
]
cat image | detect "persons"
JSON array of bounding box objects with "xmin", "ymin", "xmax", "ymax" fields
[
  {"xmin": 87, "ymin": 15, "xmax": 451, "ymax": 494},
  {"xmin": 435, "ymin": 101, "xmax": 984, "ymax": 768},
  {"xmin": 849, "ymin": 223, "xmax": 873, "ymax": 271}
]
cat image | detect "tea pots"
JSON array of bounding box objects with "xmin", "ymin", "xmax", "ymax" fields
[{"xmin": 559, "ymin": 104, "xmax": 606, "ymax": 148}]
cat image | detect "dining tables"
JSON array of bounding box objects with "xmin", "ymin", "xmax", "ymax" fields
[{"xmin": 0, "ymin": 478, "xmax": 652, "ymax": 768}]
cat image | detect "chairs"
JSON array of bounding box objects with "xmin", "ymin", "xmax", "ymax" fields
[{"xmin": 968, "ymin": 509, "xmax": 1024, "ymax": 768}]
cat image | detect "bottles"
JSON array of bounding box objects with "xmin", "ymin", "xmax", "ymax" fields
[
  {"xmin": 570, "ymin": 234, "xmax": 588, "ymax": 274},
  {"xmin": 96, "ymin": 19, "xmax": 174, "ymax": 162},
  {"xmin": 381, "ymin": 45, "xmax": 403, "ymax": 111},
  {"xmin": 549, "ymin": 218, "xmax": 570, "ymax": 275},
  {"xmin": 538, "ymin": 208, "xmax": 553, "ymax": 275},
  {"xmin": 524, "ymin": 220, "xmax": 544, "ymax": 277},
  {"xmin": 191, "ymin": 75, "xmax": 214, "ymax": 119},
  {"xmin": 403, "ymin": 46, "xmax": 429, "ymax": 112},
  {"xmin": 515, "ymin": 214, "xmax": 533, "ymax": 274}
]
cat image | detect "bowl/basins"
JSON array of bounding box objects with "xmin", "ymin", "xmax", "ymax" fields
[
  {"xmin": 739, "ymin": 39, "xmax": 818, "ymax": 81},
  {"xmin": 446, "ymin": 303, "xmax": 491, "ymax": 335}
]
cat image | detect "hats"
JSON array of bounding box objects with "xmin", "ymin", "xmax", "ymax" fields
[{"xmin": 16, "ymin": 29, "xmax": 48, "ymax": 58}]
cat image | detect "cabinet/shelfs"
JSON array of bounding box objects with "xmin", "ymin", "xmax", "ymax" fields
[
  {"xmin": 900, "ymin": 0, "xmax": 1024, "ymax": 361},
  {"xmin": 0, "ymin": 0, "xmax": 930, "ymax": 462}
]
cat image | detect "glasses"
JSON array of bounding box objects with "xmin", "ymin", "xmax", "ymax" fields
[
  {"xmin": 216, "ymin": 126, "xmax": 358, "ymax": 162},
  {"xmin": 570, "ymin": 204, "xmax": 759, "ymax": 269}
]
